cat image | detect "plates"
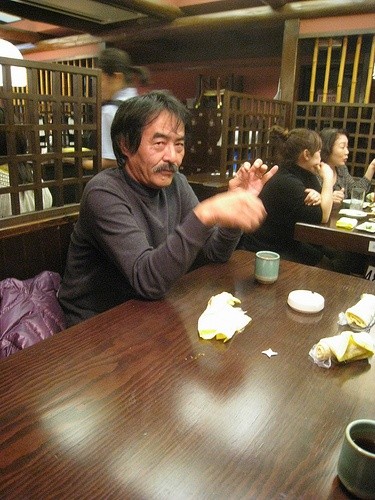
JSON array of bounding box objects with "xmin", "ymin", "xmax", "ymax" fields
[{"xmin": 338, "ymin": 209, "xmax": 367, "ymax": 220}]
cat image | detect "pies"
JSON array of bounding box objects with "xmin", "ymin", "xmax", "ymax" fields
[{"xmin": 315, "ymin": 292, "xmax": 375, "ymax": 364}]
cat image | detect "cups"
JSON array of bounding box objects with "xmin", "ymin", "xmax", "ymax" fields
[
  {"xmin": 255, "ymin": 251, "xmax": 281, "ymax": 285},
  {"xmin": 349, "ymin": 188, "xmax": 366, "ymax": 215},
  {"xmin": 337, "ymin": 419, "xmax": 375, "ymax": 500}
]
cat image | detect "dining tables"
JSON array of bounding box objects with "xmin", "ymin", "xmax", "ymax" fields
[
  {"xmin": 293, "ymin": 193, "xmax": 375, "ymax": 280},
  {"xmin": 1, "ymin": 249, "xmax": 375, "ymax": 499}
]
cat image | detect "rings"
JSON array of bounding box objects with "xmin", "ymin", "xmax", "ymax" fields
[{"xmin": 254, "ymin": 173, "xmax": 263, "ymax": 179}]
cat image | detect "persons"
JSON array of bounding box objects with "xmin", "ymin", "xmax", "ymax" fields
[
  {"xmin": 246, "ymin": 123, "xmax": 334, "ymax": 273},
  {"xmin": 311, "ymin": 126, "xmax": 375, "ymax": 205},
  {"xmin": 0, "ymin": 125, "xmax": 53, "ymax": 218},
  {"xmin": 60, "ymin": 46, "xmax": 155, "ymax": 171},
  {"xmin": 57, "ymin": 90, "xmax": 279, "ymax": 330}
]
